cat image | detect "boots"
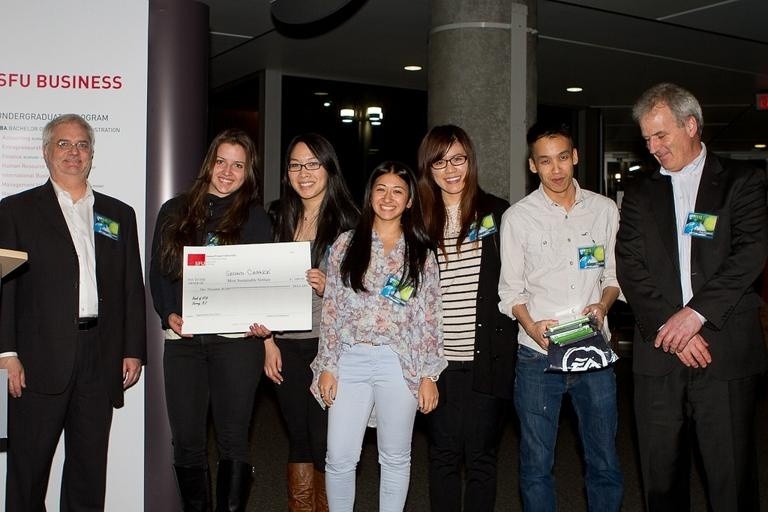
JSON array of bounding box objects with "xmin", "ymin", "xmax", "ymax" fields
[
  {"xmin": 171, "ymin": 459, "xmax": 214, "ymax": 512},
  {"xmin": 286, "ymin": 462, "xmax": 317, "ymax": 511},
  {"xmin": 215, "ymin": 457, "xmax": 258, "ymax": 511},
  {"xmin": 314, "ymin": 469, "xmax": 329, "ymax": 512}
]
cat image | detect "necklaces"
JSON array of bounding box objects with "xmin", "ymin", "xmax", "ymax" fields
[
  {"xmin": 446, "ymin": 208, "xmax": 460, "ymax": 234},
  {"xmin": 304, "ymin": 216, "xmax": 309, "ymax": 221}
]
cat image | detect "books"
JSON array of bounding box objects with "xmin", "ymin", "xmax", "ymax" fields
[{"xmin": 541, "ymin": 306, "xmax": 603, "ymax": 350}]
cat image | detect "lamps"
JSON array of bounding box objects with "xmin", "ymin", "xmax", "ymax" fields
[
  {"xmin": 342, "ymin": 117, "xmax": 353, "ymax": 123},
  {"xmin": 340, "ymin": 108, "xmax": 355, "ymax": 117},
  {"xmin": 365, "ymin": 106, "xmax": 383, "ymax": 126}
]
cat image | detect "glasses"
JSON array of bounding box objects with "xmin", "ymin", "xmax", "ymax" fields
[
  {"xmin": 431, "ymin": 155, "xmax": 467, "ymax": 170},
  {"xmin": 287, "ymin": 161, "xmax": 323, "ymax": 172},
  {"xmin": 48, "ymin": 139, "xmax": 91, "ymax": 152}
]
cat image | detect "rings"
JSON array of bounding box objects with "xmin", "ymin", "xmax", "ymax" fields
[{"xmin": 320, "ymin": 393, "xmax": 324, "ymax": 397}]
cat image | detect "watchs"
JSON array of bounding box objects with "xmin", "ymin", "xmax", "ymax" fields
[{"xmin": 424, "ymin": 374, "xmax": 442, "ymax": 383}]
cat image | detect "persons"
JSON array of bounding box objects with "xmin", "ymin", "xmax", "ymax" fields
[
  {"xmin": 410, "ymin": 125, "xmax": 517, "ymax": 512},
  {"xmin": 308, "ymin": 157, "xmax": 447, "ymax": 511},
  {"xmin": 148, "ymin": 128, "xmax": 273, "ymax": 509},
  {"xmin": 259, "ymin": 130, "xmax": 367, "ymax": 509},
  {"xmin": 610, "ymin": 79, "xmax": 767, "ymax": 512},
  {"xmin": 0, "ymin": 114, "xmax": 148, "ymax": 511},
  {"xmin": 498, "ymin": 120, "xmax": 622, "ymax": 510}
]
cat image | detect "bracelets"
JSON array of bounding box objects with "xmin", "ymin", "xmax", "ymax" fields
[{"xmin": 599, "ymin": 302, "xmax": 609, "ymax": 315}]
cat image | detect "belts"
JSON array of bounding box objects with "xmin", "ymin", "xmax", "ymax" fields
[{"xmin": 77, "ymin": 318, "xmax": 99, "ymax": 332}]
